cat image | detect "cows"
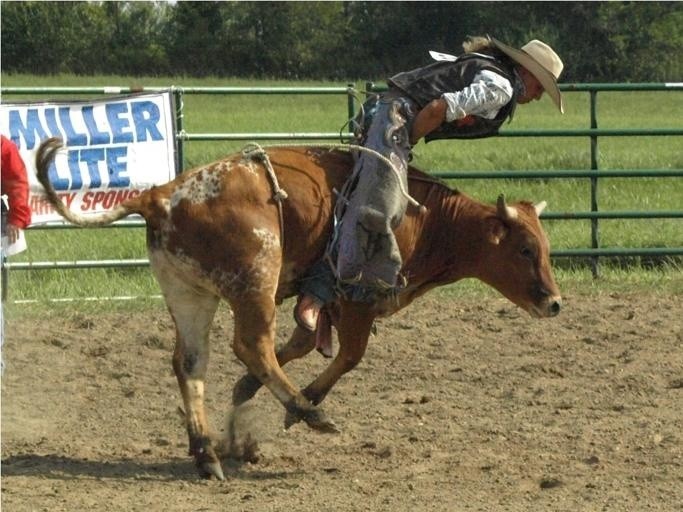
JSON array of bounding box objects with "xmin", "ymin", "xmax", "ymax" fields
[{"xmin": 35, "ymin": 137, "xmax": 565, "ymax": 482}]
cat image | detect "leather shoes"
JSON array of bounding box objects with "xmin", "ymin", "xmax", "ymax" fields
[{"xmin": 292, "ymin": 289, "xmax": 326, "ymax": 334}]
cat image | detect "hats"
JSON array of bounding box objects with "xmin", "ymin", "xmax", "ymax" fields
[{"xmin": 492, "ymin": 36, "xmax": 565, "ymax": 115}]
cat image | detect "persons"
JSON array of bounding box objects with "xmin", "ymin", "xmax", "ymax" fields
[
  {"xmin": 0, "ymin": 135, "xmax": 31, "ymax": 377},
  {"xmin": 290, "ymin": 37, "xmax": 564, "ymax": 334}
]
